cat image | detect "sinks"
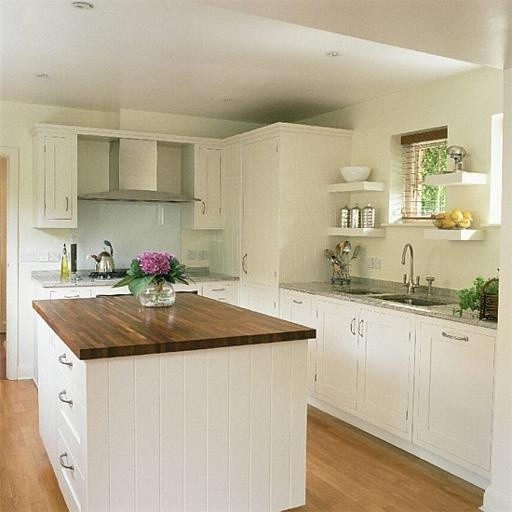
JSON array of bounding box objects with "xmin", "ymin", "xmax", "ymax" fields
[{"xmin": 367, "ymin": 294, "xmax": 452, "ymax": 306}]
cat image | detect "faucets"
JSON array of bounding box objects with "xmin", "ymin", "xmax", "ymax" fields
[{"xmin": 401, "ymin": 241, "xmax": 420, "ymax": 294}]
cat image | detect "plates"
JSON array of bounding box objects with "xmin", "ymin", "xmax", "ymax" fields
[{"xmin": 433, "ymin": 219, "xmax": 473, "ymax": 230}]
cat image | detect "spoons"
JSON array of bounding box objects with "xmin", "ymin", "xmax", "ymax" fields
[{"xmin": 342, "ymin": 240, "xmax": 351, "ymax": 263}]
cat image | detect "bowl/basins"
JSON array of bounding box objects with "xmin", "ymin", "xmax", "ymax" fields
[{"xmin": 340, "ymin": 166, "xmax": 372, "ymax": 181}]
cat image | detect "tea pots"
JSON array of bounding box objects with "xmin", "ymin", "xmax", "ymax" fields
[{"xmin": 91, "ymin": 240, "xmax": 115, "ymax": 274}]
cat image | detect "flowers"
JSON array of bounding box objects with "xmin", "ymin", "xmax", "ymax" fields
[{"xmin": 113, "ymin": 251, "xmax": 196, "ymax": 293}]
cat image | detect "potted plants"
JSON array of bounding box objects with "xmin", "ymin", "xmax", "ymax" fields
[{"xmin": 455, "ymin": 278, "xmax": 499, "ymax": 322}]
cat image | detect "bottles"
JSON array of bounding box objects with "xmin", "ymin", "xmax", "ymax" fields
[{"xmin": 60, "ymin": 244, "xmax": 69, "ymax": 276}]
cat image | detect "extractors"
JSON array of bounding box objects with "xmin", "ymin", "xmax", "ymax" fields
[{"xmin": 78, "ymin": 138, "xmax": 194, "ymax": 204}]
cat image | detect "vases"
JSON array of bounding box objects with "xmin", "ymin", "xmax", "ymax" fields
[{"xmin": 135, "ymin": 283, "xmax": 176, "ymax": 307}]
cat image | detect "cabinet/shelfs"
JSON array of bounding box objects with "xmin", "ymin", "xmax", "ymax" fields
[
  {"xmin": 239, "ymin": 137, "xmax": 279, "ymax": 318},
  {"xmin": 31, "ymin": 132, "xmax": 78, "ymax": 228},
  {"xmin": 181, "ymin": 145, "xmax": 225, "ymax": 231},
  {"xmin": 201, "ymin": 284, "xmax": 239, "ymax": 307},
  {"xmin": 315, "ymin": 301, "xmax": 416, "ymax": 444},
  {"xmin": 45, "ymin": 288, "xmax": 93, "ymax": 300},
  {"xmin": 37, "ymin": 313, "xmax": 87, "ymax": 512},
  {"xmin": 283, "ymin": 293, "xmax": 312, "ymax": 397},
  {"xmin": 428, "ymin": 324, "xmax": 496, "ymax": 473}
]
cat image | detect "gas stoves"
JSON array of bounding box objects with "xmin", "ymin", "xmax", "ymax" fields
[{"xmin": 87, "ymin": 270, "xmax": 129, "ymax": 282}]
